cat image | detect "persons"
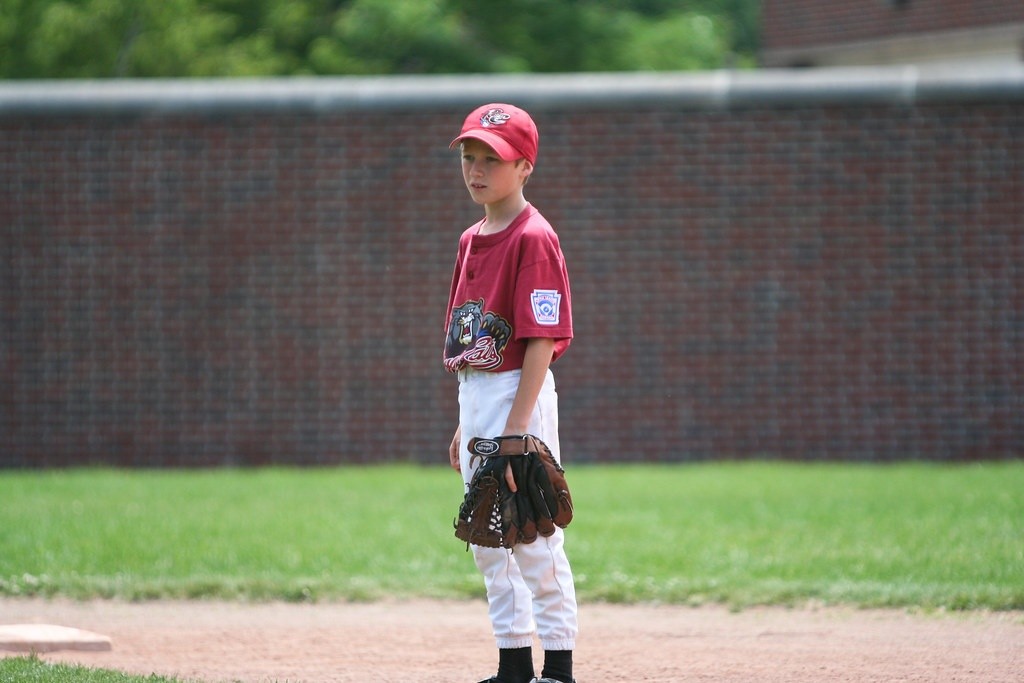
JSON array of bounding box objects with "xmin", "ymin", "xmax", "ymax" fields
[{"xmin": 443, "ymin": 102, "xmax": 574, "ymax": 683}]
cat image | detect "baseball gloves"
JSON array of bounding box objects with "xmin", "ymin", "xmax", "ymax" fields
[{"xmin": 451, "ymin": 433, "xmax": 575, "ymax": 559}]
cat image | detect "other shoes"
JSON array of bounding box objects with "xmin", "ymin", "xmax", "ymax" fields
[{"xmin": 535, "ymin": 677, "xmax": 575, "ymax": 683}]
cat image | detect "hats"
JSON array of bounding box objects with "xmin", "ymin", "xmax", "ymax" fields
[{"xmin": 449, "ymin": 103, "xmax": 538, "ymax": 168}]
